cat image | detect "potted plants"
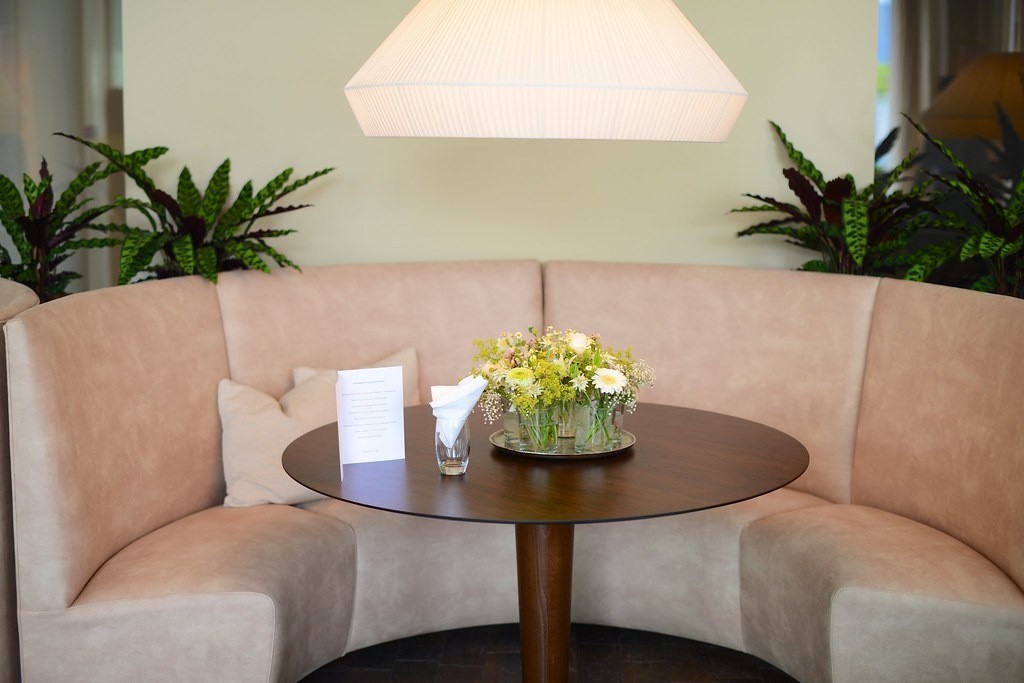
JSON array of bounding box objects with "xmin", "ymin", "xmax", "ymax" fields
[
  {"xmin": 0, "ymin": 157, "xmax": 122, "ymax": 304},
  {"xmin": 52, "ymin": 132, "xmax": 335, "ymax": 288}
]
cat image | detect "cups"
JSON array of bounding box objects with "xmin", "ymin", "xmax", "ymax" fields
[{"xmin": 434, "ymin": 417, "xmax": 470, "ymax": 475}]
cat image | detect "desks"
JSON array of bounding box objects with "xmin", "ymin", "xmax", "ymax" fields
[{"xmin": 282, "ymin": 402, "xmax": 809, "ymax": 683}]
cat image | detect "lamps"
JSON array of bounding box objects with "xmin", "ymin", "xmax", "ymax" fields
[{"xmin": 344, "ymin": 0, "xmax": 745, "ymax": 144}]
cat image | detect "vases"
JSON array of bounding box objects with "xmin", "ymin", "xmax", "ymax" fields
[{"xmin": 501, "ymin": 400, "xmax": 625, "ymax": 453}]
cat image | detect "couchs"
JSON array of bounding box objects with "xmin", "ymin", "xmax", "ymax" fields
[{"xmin": 2, "ymin": 265, "xmax": 1024, "ymax": 681}]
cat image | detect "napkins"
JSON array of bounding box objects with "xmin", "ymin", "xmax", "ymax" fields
[{"xmin": 429, "ymin": 374, "xmax": 489, "ymax": 447}]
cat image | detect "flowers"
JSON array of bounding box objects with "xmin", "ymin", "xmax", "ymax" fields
[{"xmin": 470, "ymin": 324, "xmax": 656, "ymax": 412}]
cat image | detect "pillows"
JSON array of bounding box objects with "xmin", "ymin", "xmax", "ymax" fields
[
  {"xmin": 217, "ymin": 369, "xmax": 338, "ymax": 506},
  {"xmin": 294, "ymin": 348, "xmax": 421, "ymax": 407}
]
